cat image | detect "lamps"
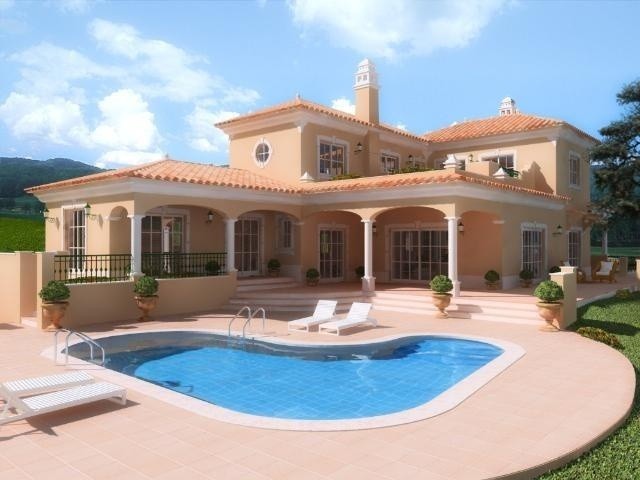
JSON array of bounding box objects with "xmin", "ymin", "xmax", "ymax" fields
[
  {"xmin": 553, "ymin": 225, "xmax": 564, "ymax": 237},
  {"xmin": 407, "ymin": 154, "xmax": 413, "ymax": 164},
  {"xmin": 468, "ymin": 152, "xmax": 474, "ymax": 162},
  {"xmin": 42, "ymin": 207, "xmax": 55, "ymax": 224},
  {"xmin": 372, "ymin": 224, "xmax": 378, "ymax": 236},
  {"xmin": 84, "ymin": 202, "xmax": 98, "ymax": 221},
  {"xmin": 205, "ymin": 209, "xmax": 214, "ymax": 224},
  {"xmin": 354, "ymin": 141, "xmax": 363, "ymax": 154},
  {"xmin": 457, "ymin": 221, "xmax": 465, "ymax": 235}
]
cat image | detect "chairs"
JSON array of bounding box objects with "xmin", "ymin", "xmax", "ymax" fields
[
  {"xmin": 561, "ymin": 260, "xmax": 585, "ymax": 283},
  {"xmin": 286, "ymin": 298, "xmax": 340, "ymax": 334},
  {"xmin": 319, "ymin": 301, "xmax": 378, "ymax": 335},
  {"xmin": 0, "ymin": 380, "xmax": 128, "ymax": 425},
  {"xmin": 590, "ymin": 257, "xmax": 620, "ymax": 284},
  {"xmin": 0, "ymin": 369, "xmax": 95, "ymax": 400}
]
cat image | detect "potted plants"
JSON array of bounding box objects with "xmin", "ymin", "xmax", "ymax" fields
[
  {"xmin": 37, "ymin": 279, "xmax": 72, "ymax": 334},
  {"xmin": 534, "ymin": 281, "xmax": 564, "ymax": 333},
  {"xmin": 267, "ymin": 258, "xmax": 281, "ymax": 276},
  {"xmin": 484, "ymin": 270, "xmax": 503, "ymax": 290},
  {"xmin": 132, "ymin": 277, "xmax": 160, "ymax": 322},
  {"xmin": 518, "ymin": 268, "xmax": 533, "ymax": 288},
  {"xmin": 204, "ymin": 260, "xmax": 221, "ymax": 276},
  {"xmin": 305, "ymin": 267, "xmax": 321, "ymax": 287},
  {"xmin": 428, "ymin": 275, "xmax": 453, "ymax": 321}
]
